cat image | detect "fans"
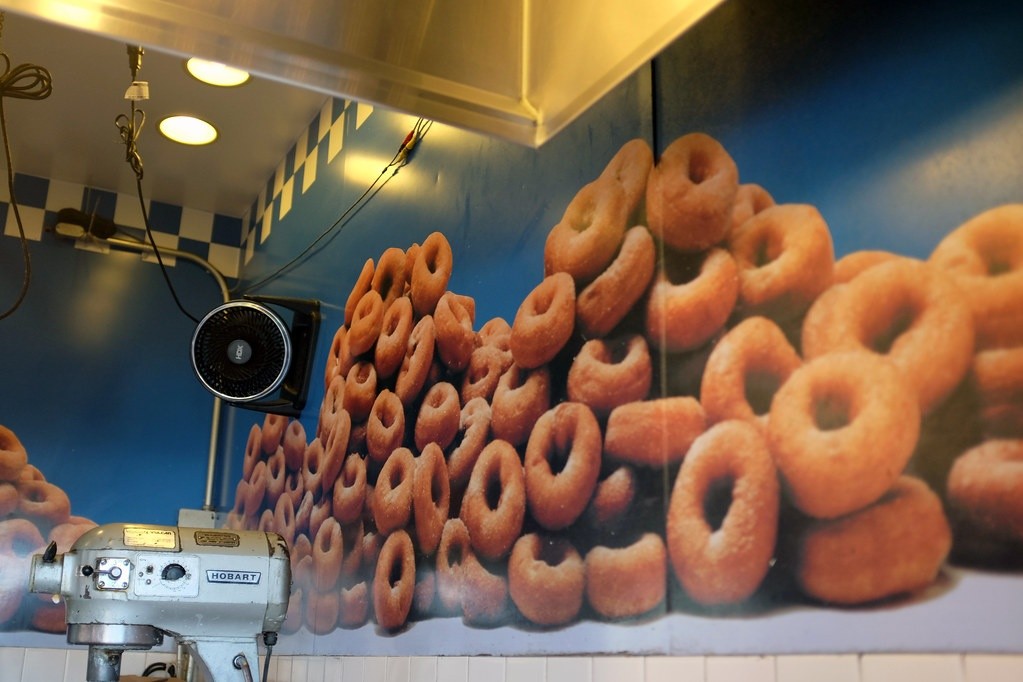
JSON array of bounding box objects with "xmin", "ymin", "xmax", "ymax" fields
[{"xmin": 190, "ymin": 294, "xmax": 322, "ymax": 417}]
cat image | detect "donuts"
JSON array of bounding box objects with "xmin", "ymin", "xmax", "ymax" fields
[
  {"xmin": 223, "ymin": 128, "xmax": 1023, "ymax": 634},
  {"xmin": 0, "ymin": 426, "xmax": 105, "ymax": 635}
]
